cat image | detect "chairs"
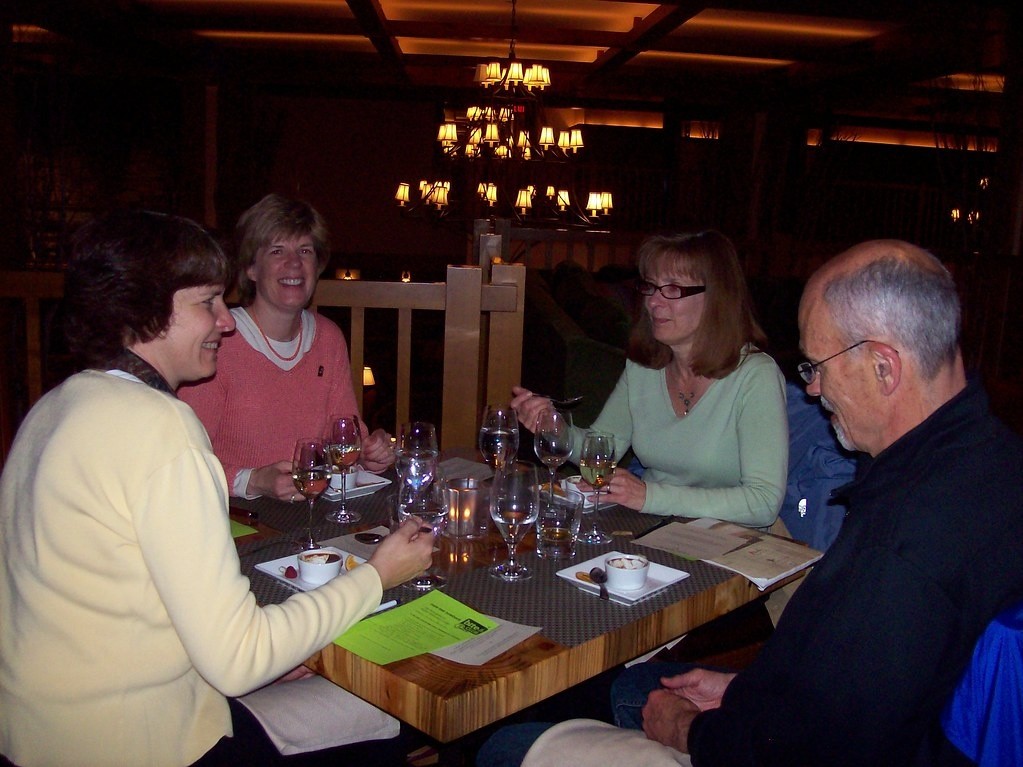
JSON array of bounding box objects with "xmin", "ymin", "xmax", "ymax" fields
[
  {"xmin": 626, "ymin": 387, "xmax": 856, "ymax": 668},
  {"xmin": 918, "ymin": 602, "xmax": 1023, "ymax": 767}
]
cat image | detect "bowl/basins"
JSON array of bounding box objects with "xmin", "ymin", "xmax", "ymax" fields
[
  {"xmin": 566, "ymin": 476, "xmax": 595, "ymax": 505},
  {"xmin": 330, "ymin": 468, "xmax": 358, "ymax": 491},
  {"xmin": 604, "ymin": 555, "xmax": 650, "ymax": 591},
  {"xmin": 297, "ymin": 550, "xmax": 342, "ymax": 585}
]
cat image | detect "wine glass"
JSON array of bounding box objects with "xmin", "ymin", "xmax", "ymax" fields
[
  {"xmin": 490, "ymin": 459, "xmax": 540, "ymax": 581},
  {"xmin": 322, "ymin": 414, "xmax": 362, "ymax": 523},
  {"xmin": 292, "ymin": 437, "xmax": 333, "ymax": 553},
  {"xmin": 398, "ymin": 472, "xmax": 449, "ymax": 590},
  {"xmin": 577, "ymin": 431, "xmax": 617, "ymax": 544},
  {"xmin": 533, "ymin": 408, "xmax": 574, "ymax": 504}
]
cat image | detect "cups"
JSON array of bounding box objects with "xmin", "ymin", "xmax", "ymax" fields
[
  {"xmin": 479, "ymin": 403, "xmax": 520, "ymax": 473},
  {"xmin": 533, "ymin": 489, "xmax": 585, "ymax": 560},
  {"xmin": 442, "ymin": 478, "xmax": 486, "ymax": 536},
  {"xmin": 396, "ymin": 422, "xmax": 438, "ymax": 481},
  {"xmin": 386, "ymin": 494, "xmax": 398, "ymax": 526}
]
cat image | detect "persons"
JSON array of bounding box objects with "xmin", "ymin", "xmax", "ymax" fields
[
  {"xmin": 475, "ymin": 238, "xmax": 1023, "ymax": 767},
  {"xmin": 508, "ymin": 224, "xmax": 791, "ymax": 727},
  {"xmin": 0, "ymin": 208, "xmax": 551, "ymax": 767},
  {"xmin": 542, "ymin": 258, "xmax": 674, "ymax": 371},
  {"xmin": 174, "ymin": 191, "xmax": 398, "ymax": 503}
]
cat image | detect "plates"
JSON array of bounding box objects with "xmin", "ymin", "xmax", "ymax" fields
[
  {"xmin": 253, "ymin": 546, "xmax": 367, "ymax": 591},
  {"xmin": 555, "ymin": 551, "xmax": 690, "ymax": 607},
  {"xmin": 531, "ymin": 478, "xmax": 603, "ymax": 509},
  {"xmin": 321, "ymin": 471, "xmax": 392, "ymax": 503}
]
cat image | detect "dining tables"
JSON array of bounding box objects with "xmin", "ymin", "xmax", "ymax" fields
[{"xmin": 230, "ymin": 442, "xmax": 815, "ymax": 746}]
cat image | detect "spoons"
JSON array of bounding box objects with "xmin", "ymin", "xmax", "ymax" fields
[
  {"xmin": 354, "ymin": 527, "xmax": 432, "ymax": 546},
  {"xmin": 590, "ymin": 567, "xmax": 609, "ymax": 601},
  {"xmin": 532, "ymin": 393, "xmax": 584, "ymax": 404}
]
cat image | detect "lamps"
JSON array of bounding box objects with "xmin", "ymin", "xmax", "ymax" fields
[
  {"xmin": 823, "ymin": 116, "xmax": 1003, "ymax": 161},
  {"xmin": 586, "ymin": 102, "xmax": 666, "ymax": 133},
  {"xmin": 917, "ymin": 66, "xmax": 1006, "ymax": 96},
  {"xmin": 388, "ymin": 0, "xmax": 622, "ymax": 230}
]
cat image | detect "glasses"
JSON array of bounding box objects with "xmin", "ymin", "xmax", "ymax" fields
[
  {"xmin": 796, "ymin": 340, "xmax": 899, "ymax": 385},
  {"xmin": 635, "ymin": 280, "xmax": 707, "ymax": 301}
]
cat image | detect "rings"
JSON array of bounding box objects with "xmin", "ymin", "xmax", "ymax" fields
[
  {"xmin": 607, "ymin": 483, "xmax": 611, "ymax": 494},
  {"xmin": 387, "ymin": 445, "xmax": 395, "ymax": 453},
  {"xmin": 290, "ymin": 495, "xmax": 295, "ymax": 504}
]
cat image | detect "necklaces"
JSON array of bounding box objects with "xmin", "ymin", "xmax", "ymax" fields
[
  {"xmin": 669, "ymin": 365, "xmax": 702, "ymax": 416},
  {"xmin": 251, "ymin": 302, "xmax": 303, "ymax": 361}
]
